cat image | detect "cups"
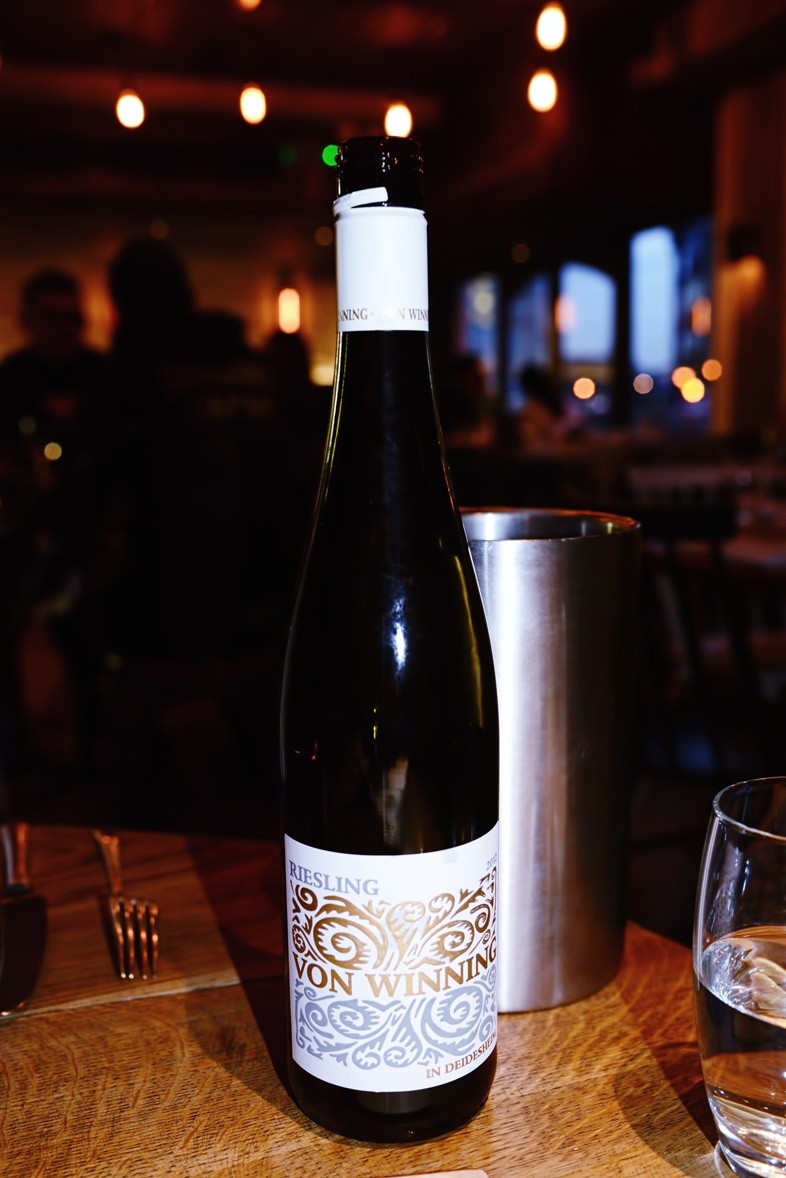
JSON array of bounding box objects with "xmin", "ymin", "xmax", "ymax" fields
[
  {"xmin": 462, "ymin": 508, "xmax": 640, "ymax": 1012},
  {"xmin": 692, "ymin": 774, "xmax": 786, "ymax": 1176}
]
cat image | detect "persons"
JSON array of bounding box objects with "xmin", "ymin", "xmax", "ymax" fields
[
  {"xmin": 1, "ymin": 262, "xmax": 105, "ymax": 427},
  {"xmin": 431, "ymin": 351, "xmax": 526, "ymax": 464},
  {"xmin": 516, "ymin": 360, "xmax": 591, "ymax": 446},
  {"xmin": 78, "ymin": 231, "xmax": 333, "ymax": 552}
]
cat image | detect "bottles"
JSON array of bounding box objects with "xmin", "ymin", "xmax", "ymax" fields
[{"xmin": 282, "ymin": 136, "xmax": 497, "ymax": 1141}]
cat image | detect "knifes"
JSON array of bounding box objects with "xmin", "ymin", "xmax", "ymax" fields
[{"xmin": 1, "ymin": 822, "xmax": 44, "ymax": 1013}]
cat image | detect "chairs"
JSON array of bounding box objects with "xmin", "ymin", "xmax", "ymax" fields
[{"xmin": 612, "ymin": 474, "xmax": 786, "ymax": 847}]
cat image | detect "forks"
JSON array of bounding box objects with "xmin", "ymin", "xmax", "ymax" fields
[{"xmin": 94, "ymin": 833, "xmax": 161, "ymax": 980}]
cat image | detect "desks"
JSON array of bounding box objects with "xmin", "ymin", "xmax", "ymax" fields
[{"xmin": 0, "ymin": 822, "xmax": 743, "ymax": 1178}]
